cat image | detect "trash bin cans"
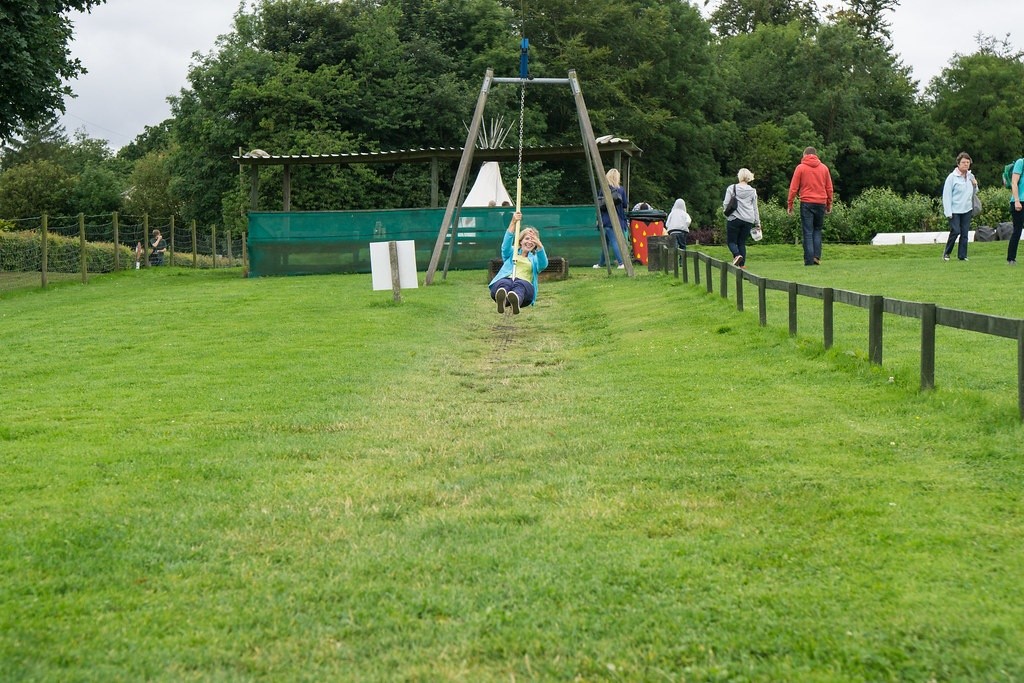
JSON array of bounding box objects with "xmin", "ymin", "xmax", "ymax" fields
[{"xmin": 628, "ymin": 209, "xmax": 668, "ymax": 267}]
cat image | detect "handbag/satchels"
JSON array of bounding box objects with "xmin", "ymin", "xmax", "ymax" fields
[
  {"xmin": 724, "ymin": 183, "xmax": 738, "ymax": 217},
  {"xmin": 972, "ymin": 186, "xmax": 982, "ymax": 218},
  {"xmin": 974, "ymin": 226, "xmax": 997, "ymax": 242},
  {"xmin": 750, "ymin": 227, "xmax": 763, "ymax": 242},
  {"xmin": 597, "ymin": 185, "xmax": 623, "ymax": 212},
  {"xmin": 997, "ymin": 222, "xmax": 1014, "ymax": 240}
]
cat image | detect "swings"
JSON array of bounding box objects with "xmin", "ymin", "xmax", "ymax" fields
[{"xmin": 493, "ymin": 84, "xmax": 543, "ymax": 311}]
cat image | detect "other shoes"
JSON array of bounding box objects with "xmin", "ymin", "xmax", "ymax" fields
[
  {"xmin": 732, "ymin": 255, "xmax": 743, "ymax": 266},
  {"xmin": 740, "ymin": 266, "xmax": 745, "ymax": 269},
  {"xmin": 496, "ymin": 287, "xmax": 506, "ymax": 314},
  {"xmin": 679, "ymin": 263, "xmax": 682, "ymax": 266},
  {"xmin": 1007, "ymin": 260, "xmax": 1017, "ymax": 264},
  {"xmin": 617, "ymin": 264, "xmax": 624, "ymax": 269},
  {"xmin": 593, "ymin": 264, "xmax": 603, "ymax": 268},
  {"xmin": 507, "ymin": 291, "xmax": 521, "ymax": 315},
  {"xmin": 814, "ymin": 257, "xmax": 820, "ymax": 264},
  {"xmin": 942, "ymin": 250, "xmax": 950, "ymax": 261},
  {"xmin": 958, "ymin": 257, "xmax": 970, "ymax": 261}
]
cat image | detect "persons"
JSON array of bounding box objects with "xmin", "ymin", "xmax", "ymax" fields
[
  {"xmin": 943, "ymin": 152, "xmax": 978, "ymax": 261},
  {"xmin": 593, "ymin": 169, "xmax": 628, "ymax": 269},
  {"xmin": 723, "ymin": 168, "xmax": 761, "ymax": 269},
  {"xmin": 489, "ymin": 212, "xmax": 548, "ymax": 314},
  {"xmin": 633, "ymin": 203, "xmax": 653, "ymax": 211},
  {"xmin": 665, "ymin": 198, "xmax": 691, "ymax": 267},
  {"xmin": 788, "ymin": 147, "xmax": 834, "ymax": 265},
  {"xmin": 149, "ymin": 230, "xmax": 166, "ymax": 266},
  {"xmin": 1007, "ymin": 158, "xmax": 1024, "ymax": 263}
]
